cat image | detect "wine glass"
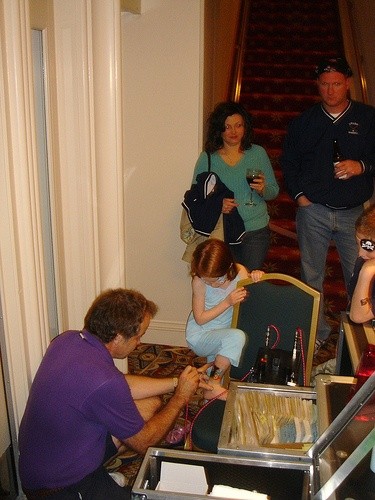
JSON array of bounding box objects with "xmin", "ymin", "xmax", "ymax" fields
[{"xmin": 244, "ymin": 168, "xmax": 263, "ymax": 206}]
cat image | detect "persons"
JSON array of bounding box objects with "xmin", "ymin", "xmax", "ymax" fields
[
  {"xmin": 18, "ymin": 289, "xmax": 214, "ymax": 500},
  {"xmin": 184, "ymin": 238, "xmax": 265, "ymax": 399},
  {"xmin": 280, "ymin": 55, "xmax": 375, "ymax": 352},
  {"xmin": 310, "ymin": 206, "xmax": 375, "ymax": 386},
  {"xmin": 191, "ymin": 101, "xmax": 279, "ymax": 272}
]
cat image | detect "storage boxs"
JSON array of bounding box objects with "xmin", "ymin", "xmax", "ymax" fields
[{"xmin": 131, "ymin": 372, "xmax": 375, "ymax": 500}]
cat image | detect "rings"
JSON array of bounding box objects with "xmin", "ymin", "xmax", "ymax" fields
[
  {"xmin": 344, "ymin": 171, "xmax": 347, "ymax": 174},
  {"xmin": 260, "ymin": 184, "xmax": 261, "ymax": 186}
]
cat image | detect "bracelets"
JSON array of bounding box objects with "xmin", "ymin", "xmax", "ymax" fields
[{"xmin": 173, "ymin": 377, "xmax": 179, "ymax": 390}]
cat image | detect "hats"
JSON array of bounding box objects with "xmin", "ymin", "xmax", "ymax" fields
[{"xmin": 315, "ymin": 53, "xmax": 352, "ymax": 79}]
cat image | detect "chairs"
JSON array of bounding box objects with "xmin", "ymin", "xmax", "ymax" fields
[{"xmin": 191, "ymin": 272, "xmax": 321, "ymax": 454}]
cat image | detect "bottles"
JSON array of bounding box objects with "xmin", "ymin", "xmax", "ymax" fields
[{"xmin": 332, "ymin": 150, "xmax": 348, "ymax": 179}]
